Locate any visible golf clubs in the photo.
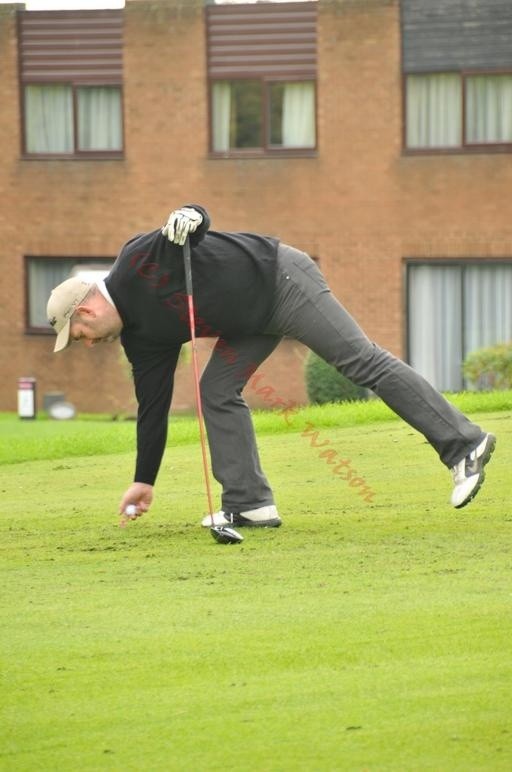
[182,233,242,543]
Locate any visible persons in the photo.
[47,203,497,532]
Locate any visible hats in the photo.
[47,277,95,353]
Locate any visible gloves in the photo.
[161,207,203,246]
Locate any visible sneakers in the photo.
[200,503,282,527]
[451,432,496,508]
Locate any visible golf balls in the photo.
[125,505,135,514]
